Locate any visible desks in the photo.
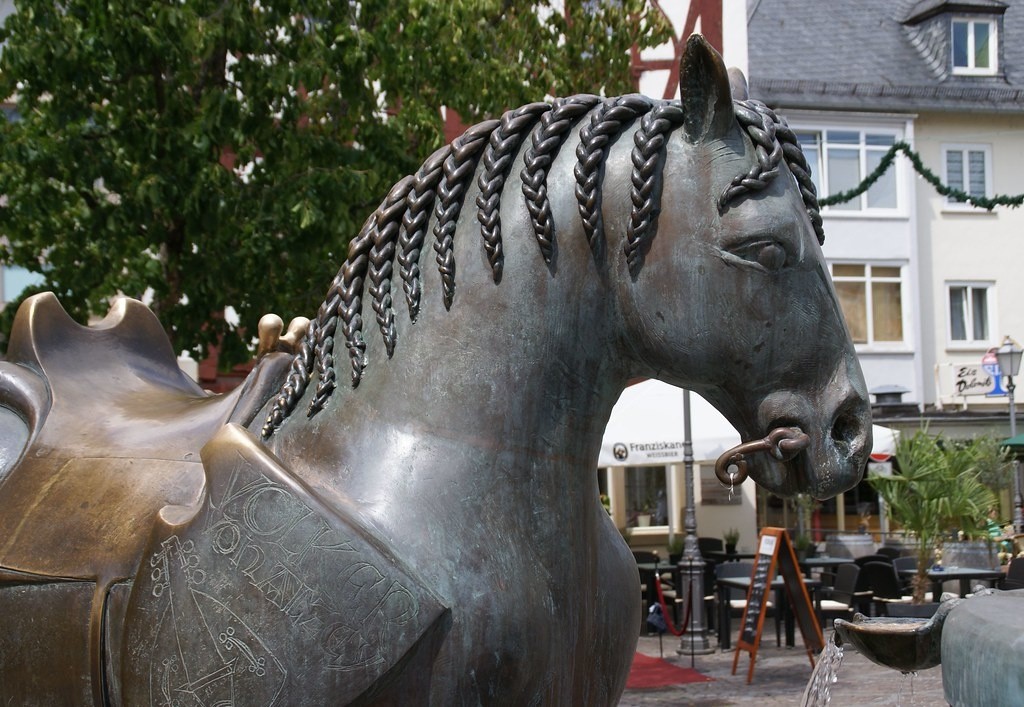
[898,565,1006,603]
[708,549,756,564]
[716,575,822,652]
[800,557,855,579]
[637,562,681,636]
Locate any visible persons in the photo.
[985,507,1015,554]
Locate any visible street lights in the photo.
[993,335,1024,540]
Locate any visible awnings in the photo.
[597,378,898,468]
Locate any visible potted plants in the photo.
[664,534,686,562]
[723,527,740,554]
[795,534,810,562]
[633,490,660,527]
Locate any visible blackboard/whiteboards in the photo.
[738,525,825,656]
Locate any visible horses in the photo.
[0,30,873,707]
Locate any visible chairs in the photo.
[631,537,1024,648]
